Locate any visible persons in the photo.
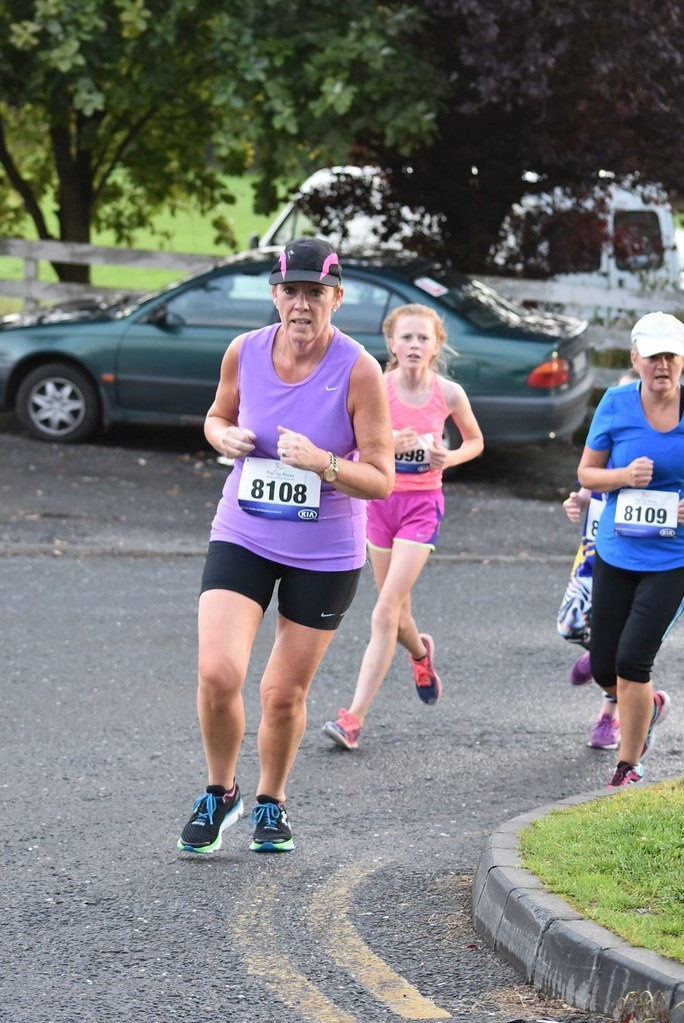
[575,312,684,788]
[176,236,397,855]
[320,303,485,750]
[555,368,643,751]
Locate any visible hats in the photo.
[269,237,342,287]
[631,310,684,358]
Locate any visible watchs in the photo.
[317,451,339,484]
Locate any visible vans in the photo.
[245,157,683,326]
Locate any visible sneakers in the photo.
[177,777,244,853]
[410,633,442,706]
[586,713,621,750]
[322,714,362,750]
[639,689,671,762]
[248,794,296,851]
[606,761,644,789]
[569,650,593,685]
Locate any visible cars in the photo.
[0,244,597,480]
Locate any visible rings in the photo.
[281,448,287,457]
